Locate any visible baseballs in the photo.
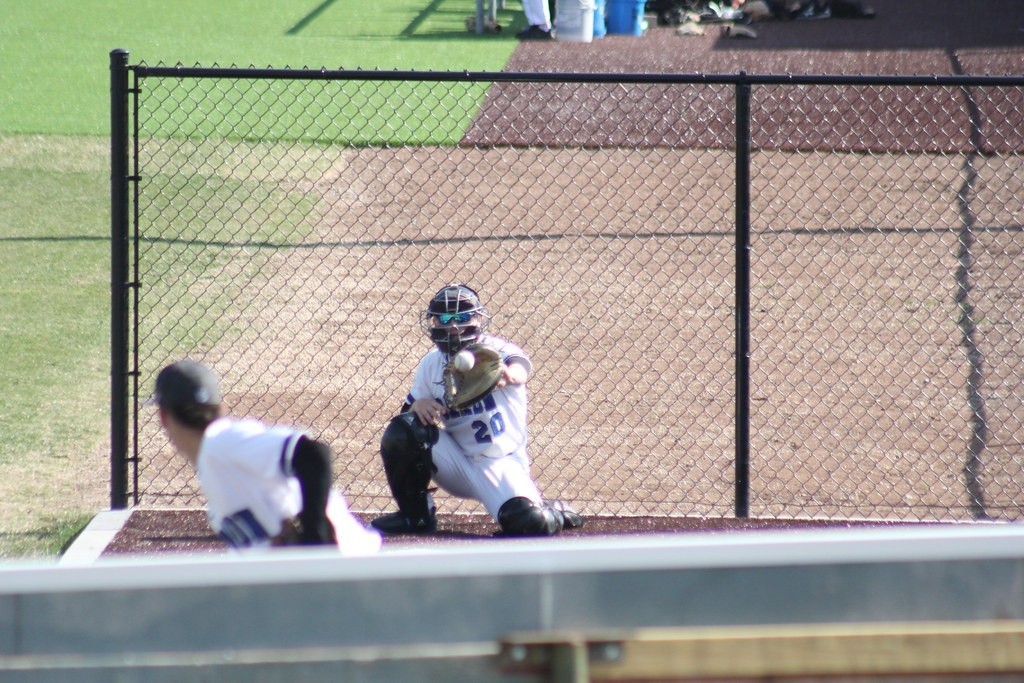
[455,349,475,372]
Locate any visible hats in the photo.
[156,361,220,410]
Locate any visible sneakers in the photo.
[550,499,583,527]
[370,511,437,533]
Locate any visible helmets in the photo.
[419,283,493,362]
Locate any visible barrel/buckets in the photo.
[552,0,648,42]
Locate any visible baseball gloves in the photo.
[441,347,503,410]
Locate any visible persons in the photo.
[513,0,554,39]
[155,360,383,551]
[369,282,584,538]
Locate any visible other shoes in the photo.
[515,25,551,38]
